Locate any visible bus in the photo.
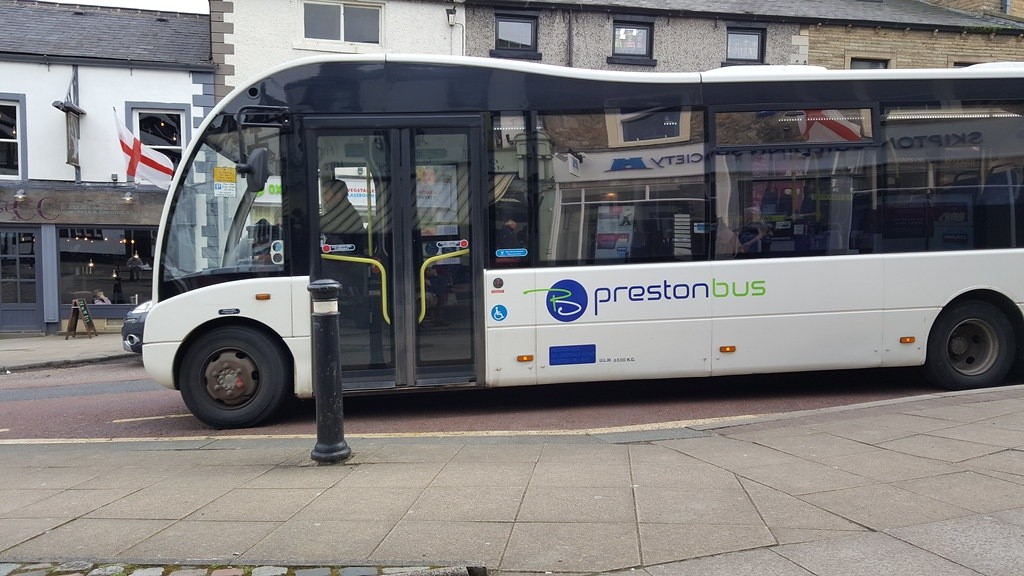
[142,54,1024,430]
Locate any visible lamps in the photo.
[122,192,134,204]
[13,189,26,203]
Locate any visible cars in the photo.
[121,300,152,354]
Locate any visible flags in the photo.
[798,110,861,143]
[114,114,174,190]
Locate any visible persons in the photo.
[318,179,382,332]
[618,208,632,227]
[663,114,674,137]
[498,219,524,258]
[728,204,772,258]
[93,289,111,305]
[426,161,474,325]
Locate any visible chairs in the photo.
[742,161,1024,259]
